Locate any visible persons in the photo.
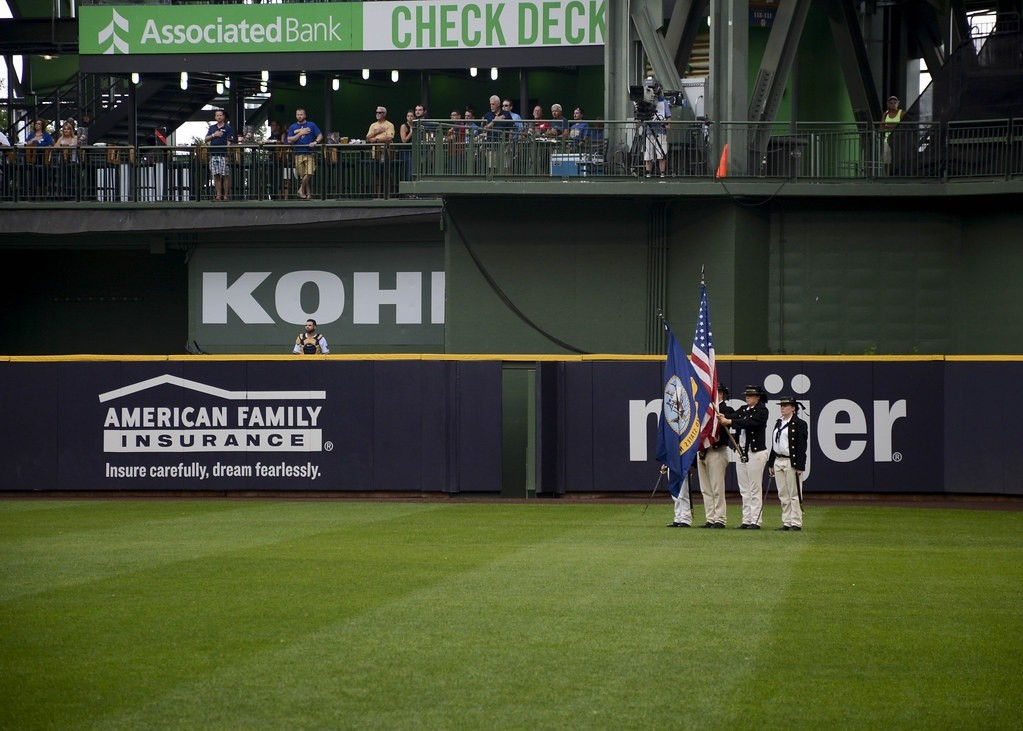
[447,95,591,178]
[292,319,330,354]
[715,385,769,529]
[643,92,672,177]
[366,106,396,199]
[205,111,236,202]
[0,118,81,200]
[288,108,323,198]
[879,95,907,176]
[696,383,736,529]
[399,104,436,197]
[767,396,808,531]
[658,463,693,528]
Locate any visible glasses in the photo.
[890,102,898,104]
[502,105,511,107]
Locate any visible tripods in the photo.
[627,117,673,175]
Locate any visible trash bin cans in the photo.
[767,137,808,177]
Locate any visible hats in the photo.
[888,96,899,102]
[741,384,762,395]
[777,396,795,405]
[717,383,726,390]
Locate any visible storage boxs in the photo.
[550,153,591,176]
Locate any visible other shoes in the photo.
[737,523,751,529]
[297,189,307,199]
[789,525,802,531]
[748,522,760,529]
[212,198,229,203]
[667,522,677,527]
[774,526,789,530]
[699,522,719,528]
[711,522,725,528]
[677,523,690,527]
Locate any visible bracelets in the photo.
[212,133,215,138]
[315,139,319,144]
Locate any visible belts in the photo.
[774,453,792,458]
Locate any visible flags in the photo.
[689,284,719,452]
[655,320,713,498]
